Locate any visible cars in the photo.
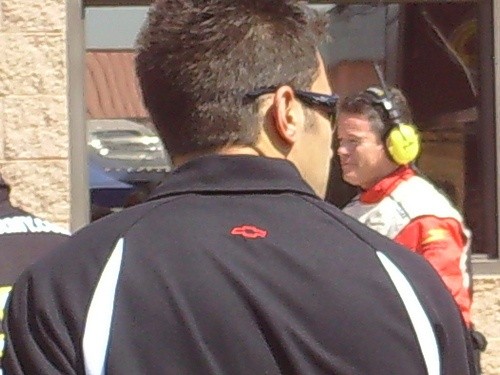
[86,117,165,171]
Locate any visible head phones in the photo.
[366,87,420,165]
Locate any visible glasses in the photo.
[245,86,338,131]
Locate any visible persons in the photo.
[1,0,481,375]
[333,79,475,331]
[0,170,72,360]
